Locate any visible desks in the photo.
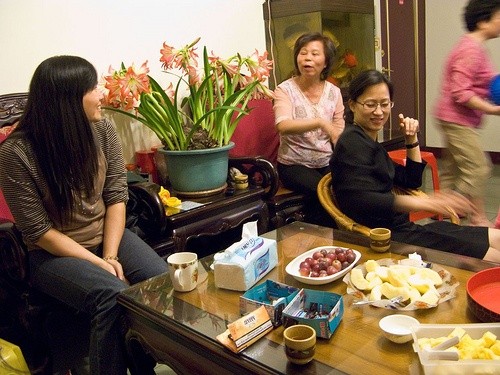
[117,222,500,375]
[164,183,270,253]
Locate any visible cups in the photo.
[166,252,198,292]
[234,175,248,189]
[369,228,391,253]
[283,325,317,365]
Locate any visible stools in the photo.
[388,149,444,222]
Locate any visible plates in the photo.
[285,245,361,284]
[342,257,460,311]
[466,267,500,323]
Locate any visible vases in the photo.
[157,142,234,197]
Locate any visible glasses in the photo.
[354,100,395,110]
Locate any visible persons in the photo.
[433,0,500,231]
[329,70,500,263]
[0,55,168,375]
[272,33,345,231]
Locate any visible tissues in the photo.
[209,220,279,291]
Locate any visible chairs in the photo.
[195,99,307,228]
[0,92,174,375]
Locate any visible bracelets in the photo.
[103,256,118,261]
[406,141,420,149]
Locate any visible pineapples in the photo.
[418,327,500,375]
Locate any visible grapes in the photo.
[299,248,356,277]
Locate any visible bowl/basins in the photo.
[412,322,500,375]
[379,313,420,344]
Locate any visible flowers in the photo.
[100,37,280,150]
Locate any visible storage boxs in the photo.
[262,0,376,110]
[240,279,344,340]
[411,322,500,375]
